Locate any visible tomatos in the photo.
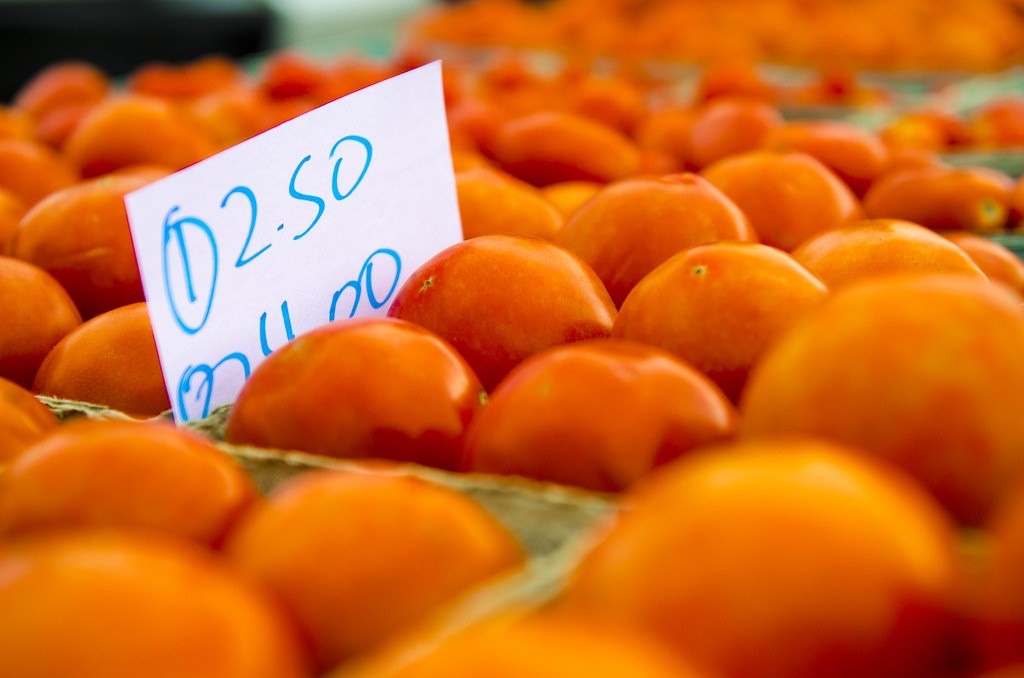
[0,0,1024,678]
